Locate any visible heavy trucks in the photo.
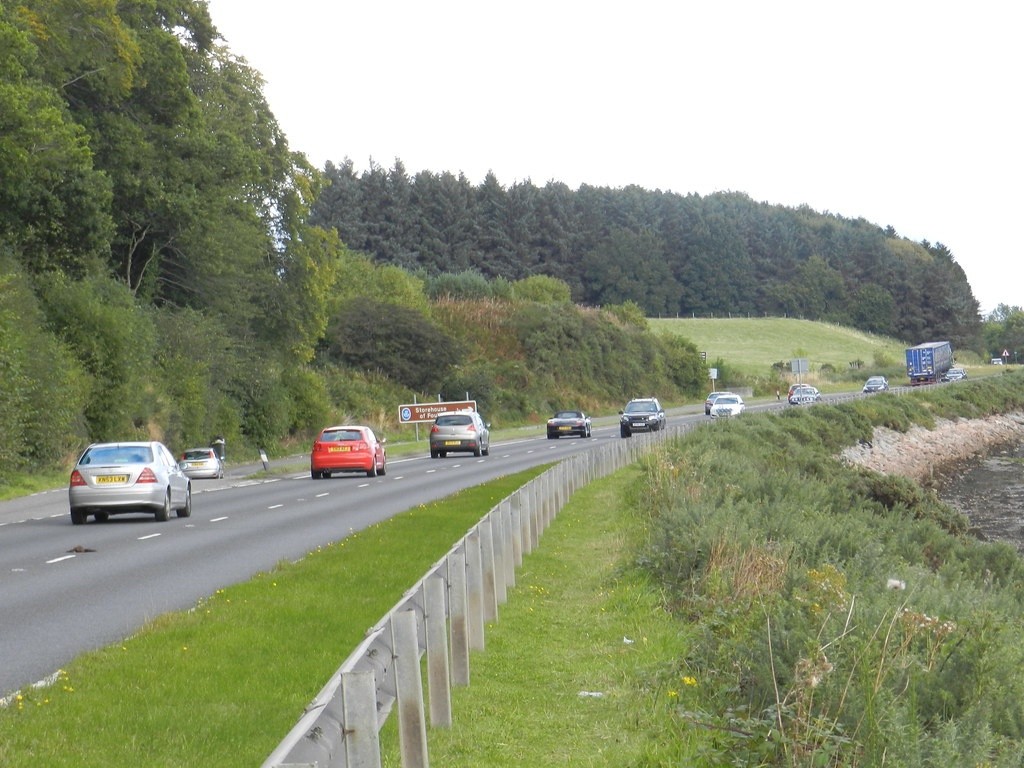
[906,340,955,387]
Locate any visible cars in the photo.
[944,368,968,382]
[709,395,746,419]
[704,391,736,415]
[788,383,824,405]
[429,411,492,459]
[179,446,224,480]
[67,440,191,525]
[546,410,593,439]
[309,424,387,479]
[862,375,889,393]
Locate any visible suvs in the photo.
[619,397,667,438]
